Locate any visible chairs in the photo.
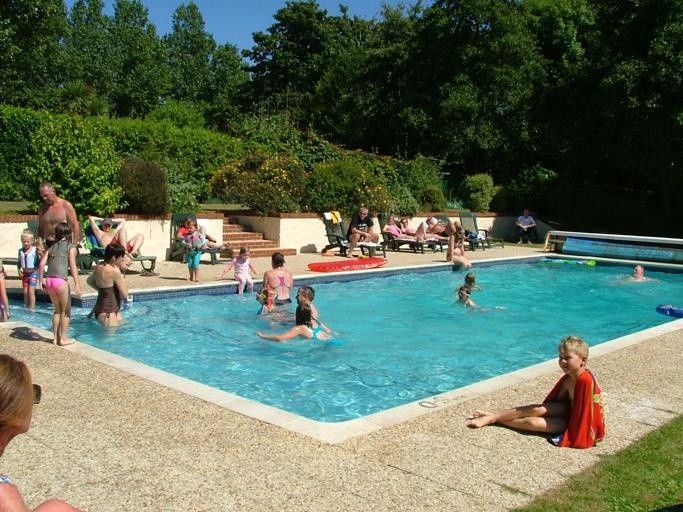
[168,212,234,265]
[21,219,100,278]
[314,206,539,259]
[77,215,157,280]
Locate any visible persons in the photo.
[456,287,473,306]
[461,273,481,291]
[447,228,471,273]
[0,261,10,324]
[628,264,646,279]
[36,182,79,258]
[0,354,82,512]
[346,206,485,257]
[467,335,605,448]
[515,210,537,244]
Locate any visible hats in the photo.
[102,218,113,225]
